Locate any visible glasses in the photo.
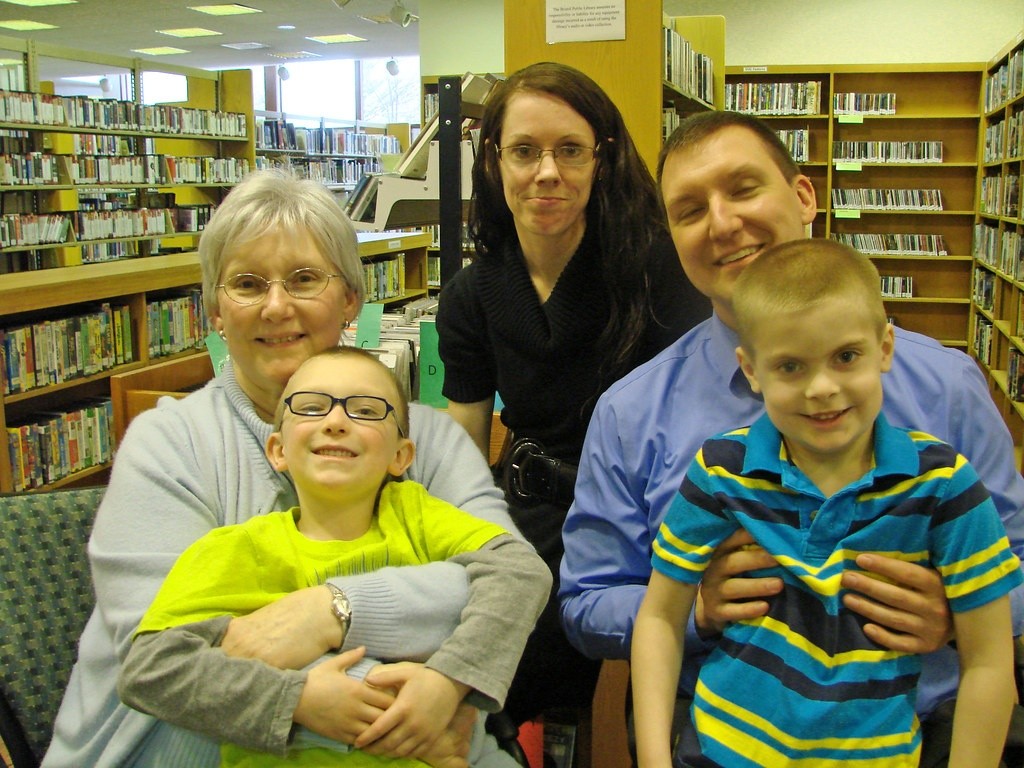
[275,392,406,437]
[493,142,600,166]
[215,267,344,304]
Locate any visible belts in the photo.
[493,429,575,506]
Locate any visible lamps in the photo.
[332,0,351,9]
[98,76,114,92]
[277,63,289,80]
[389,2,415,28]
[385,55,402,77]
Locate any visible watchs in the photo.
[325,583,352,646]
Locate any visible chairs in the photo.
[0,472,529,768]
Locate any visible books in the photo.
[662,29,1024,402]
[0,71,505,497]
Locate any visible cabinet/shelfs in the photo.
[0,0,1024,495]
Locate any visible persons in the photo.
[436,61,704,768]
[39,166,553,768]
[557,109,1024,768]
